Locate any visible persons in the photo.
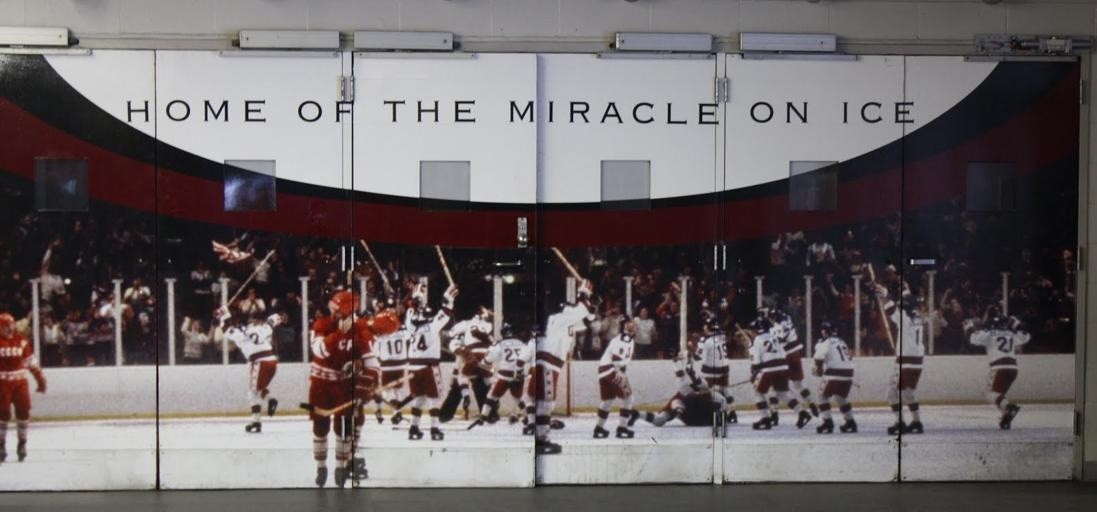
[4,206,1077,492]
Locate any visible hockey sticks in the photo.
[851,263,895,351]
[300,374,416,417]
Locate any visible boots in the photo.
[18,443,26,456]
[432,427,444,436]
[410,425,423,435]
[375,410,402,422]
[0,445,7,457]
[247,422,261,431]
[268,399,277,415]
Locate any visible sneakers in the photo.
[479,415,565,432]
[315,459,365,486]
[593,408,641,438]
[721,405,1016,434]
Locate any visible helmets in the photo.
[898,293,916,314]
[819,321,833,335]
[411,304,542,338]
[704,317,720,332]
[991,315,1007,329]
[374,311,399,337]
[0,313,15,339]
[768,306,783,322]
[618,314,636,338]
[328,290,359,319]
[751,317,772,333]
